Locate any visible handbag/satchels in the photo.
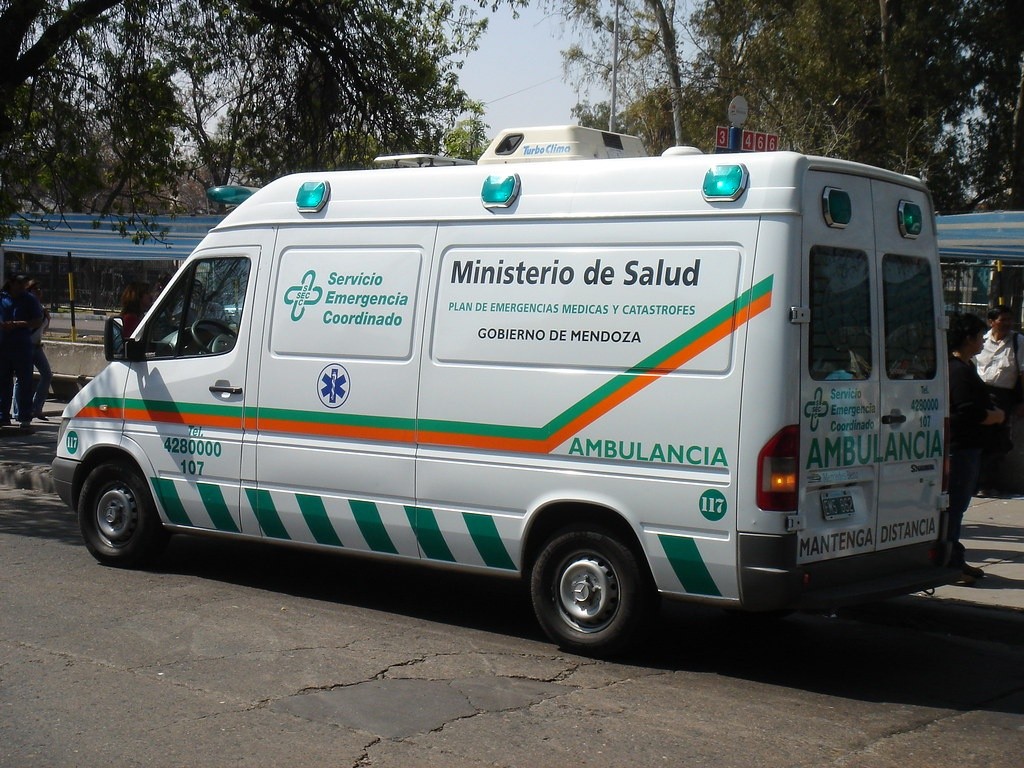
[1010,375,1024,404]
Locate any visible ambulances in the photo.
[52,128,953,661]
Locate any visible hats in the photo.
[25,280,40,289]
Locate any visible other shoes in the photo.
[0,420,11,425]
[20,422,35,434]
[32,409,49,421]
[990,489,1000,497]
[977,489,986,497]
[955,562,984,581]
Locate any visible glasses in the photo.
[35,287,41,290]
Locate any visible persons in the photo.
[120,271,195,343]
[13,277,52,421]
[970,304,1024,499]
[945,312,1006,585]
[0,272,44,434]
[818,349,870,537]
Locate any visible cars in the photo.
[220,292,246,319]
